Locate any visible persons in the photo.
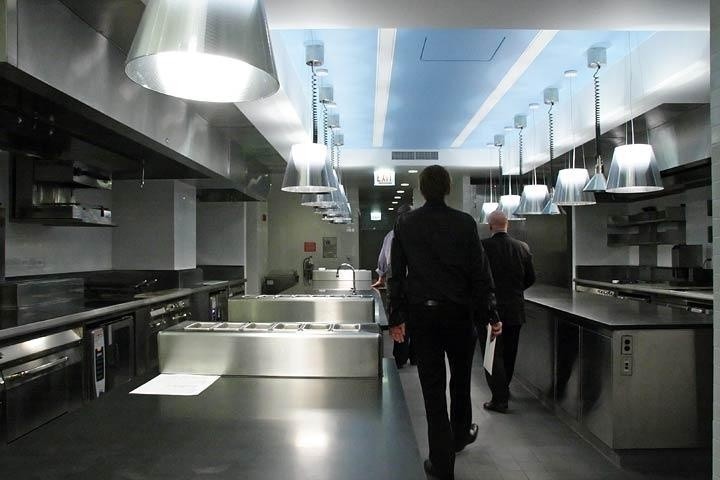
[475,207,534,416]
[384,161,504,480]
[371,204,419,369]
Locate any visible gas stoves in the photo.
[610,274,674,288]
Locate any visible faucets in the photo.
[336,262,356,292]
[136,279,158,293]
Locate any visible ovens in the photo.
[0,323,87,446]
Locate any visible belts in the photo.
[426,299,445,307]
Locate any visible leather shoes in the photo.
[454,423,479,452]
[424,458,433,476]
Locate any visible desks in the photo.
[512,282,713,474]
[0,356,431,479]
[277,281,391,356]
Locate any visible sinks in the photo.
[134,286,190,299]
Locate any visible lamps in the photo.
[122,0,282,104]
[477,46,665,225]
[279,37,338,195]
[297,83,352,227]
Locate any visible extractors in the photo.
[10,159,115,228]
[605,207,687,246]
